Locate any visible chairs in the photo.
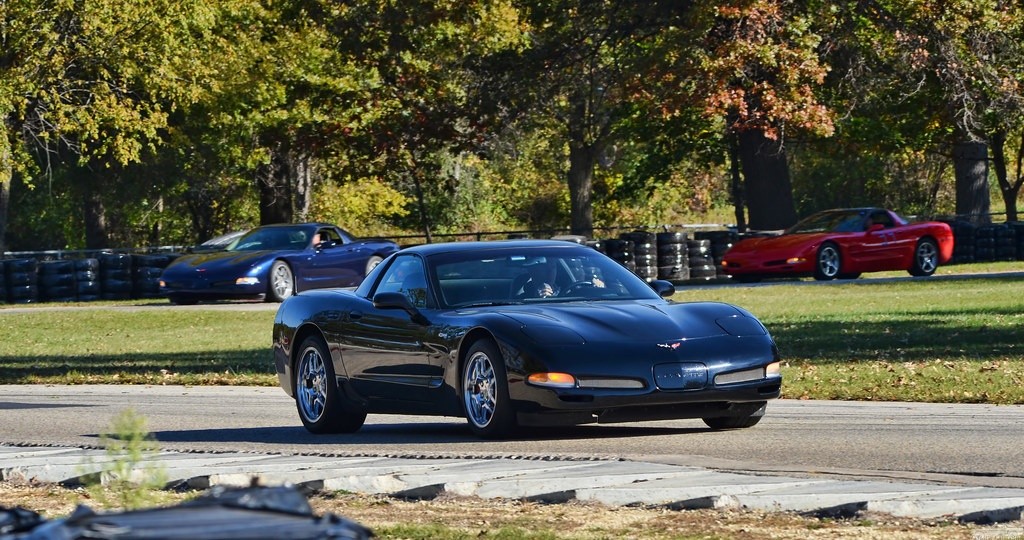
[320,231,332,242]
[400,272,428,308]
[509,273,534,299]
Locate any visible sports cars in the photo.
[158,222,401,306]
[721,207,954,281]
[272,239,783,440]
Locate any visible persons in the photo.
[517,260,605,298]
[311,233,327,249]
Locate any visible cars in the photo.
[163,231,248,261]
